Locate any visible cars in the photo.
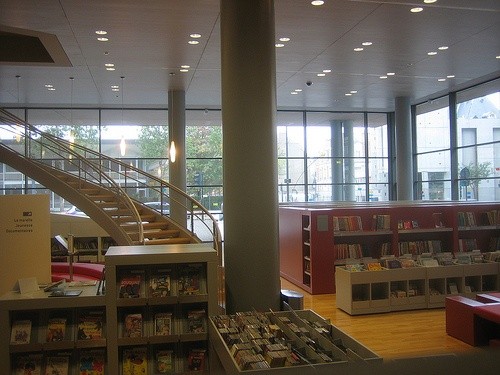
[67,205,88,216]
[144,202,170,216]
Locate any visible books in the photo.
[459,237,500,252]
[398,240,442,256]
[212,311,347,370]
[303,215,310,287]
[345,250,500,271]
[458,210,497,227]
[334,243,391,259]
[391,282,471,298]
[333,215,390,230]
[119,266,208,375]
[10,313,106,375]
[398,220,446,229]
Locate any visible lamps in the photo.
[169,73,176,163]
[69,77,75,151]
[120,76,126,155]
[16,76,21,142]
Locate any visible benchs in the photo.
[51,259,105,281]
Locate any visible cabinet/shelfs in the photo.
[0,247,217,375]
[279,204,500,295]
[208,310,383,375]
[335,258,500,316]
